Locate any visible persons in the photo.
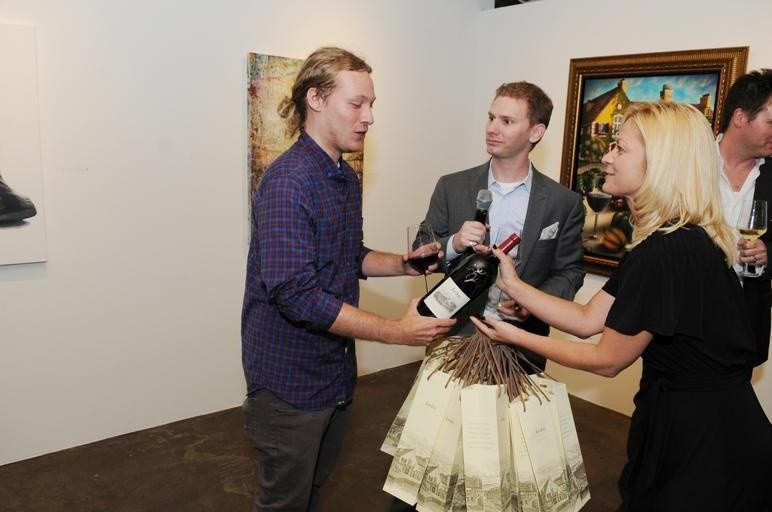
[389,80,586,510]
[469,101,771,510]
[699,67,771,382]
[242,46,458,512]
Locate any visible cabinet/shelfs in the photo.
[417,232,521,321]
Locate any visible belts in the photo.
[584,174,612,243]
[733,201,767,279]
[408,225,438,295]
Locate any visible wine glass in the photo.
[752,256,757,264]
[470,241,473,247]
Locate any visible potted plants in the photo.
[559,45,749,278]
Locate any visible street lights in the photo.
[378,335,593,512]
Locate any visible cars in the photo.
[483,257,522,319]
